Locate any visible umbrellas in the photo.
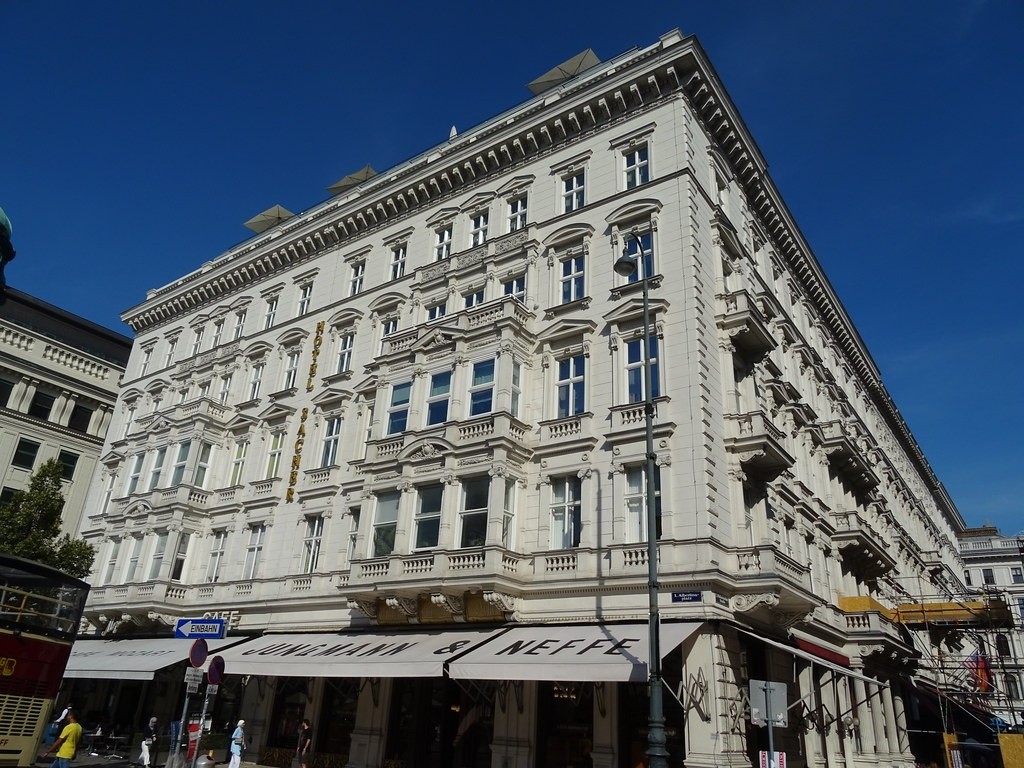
[327,164,377,194]
[524,48,600,95]
[243,204,295,233]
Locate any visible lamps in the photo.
[805,712,818,735]
[825,713,835,737]
[841,716,853,739]
[848,718,860,739]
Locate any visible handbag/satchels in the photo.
[145,738,153,745]
[235,738,242,743]
[292,752,299,768]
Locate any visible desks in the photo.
[104,736,129,760]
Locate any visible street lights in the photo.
[614,232,670,768]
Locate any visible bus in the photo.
[0,553,91,768]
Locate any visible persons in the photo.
[42,710,82,768]
[138,717,158,768]
[54,704,74,752]
[298,719,312,768]
[228,720,246,768]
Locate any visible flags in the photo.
[962,647,995,700]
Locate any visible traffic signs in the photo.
[175,619,224,639]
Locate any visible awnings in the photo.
[917,679,1013,729]
[62,620,706,711]
